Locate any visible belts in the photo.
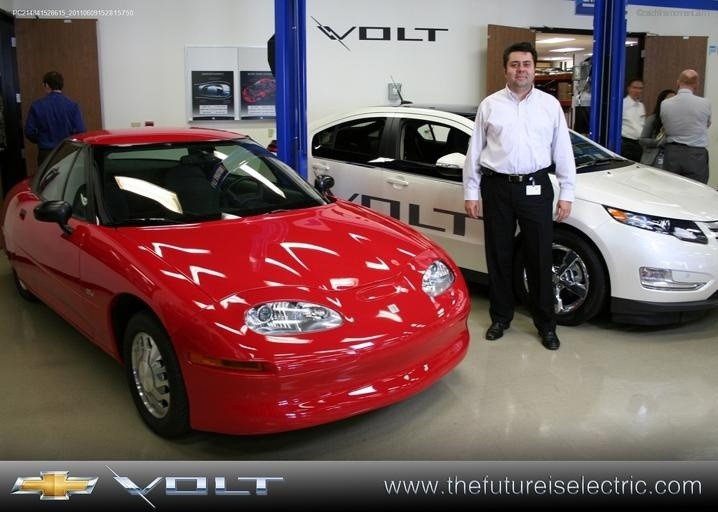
[665,142,688,147]
[480,165,548,183]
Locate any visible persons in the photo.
[24,71,84,167]
[639,70,711,185]
[463,42,576,350]
[622,78,646,162]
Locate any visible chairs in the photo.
[75,165,222,218]
[334,126,468,157]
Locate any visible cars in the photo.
[241,80,275,104]
[193,80,232,102]
[255,73,718,328]
[0,125,474,440]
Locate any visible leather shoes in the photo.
[538,328,560,350]
[486,323,510,341]
